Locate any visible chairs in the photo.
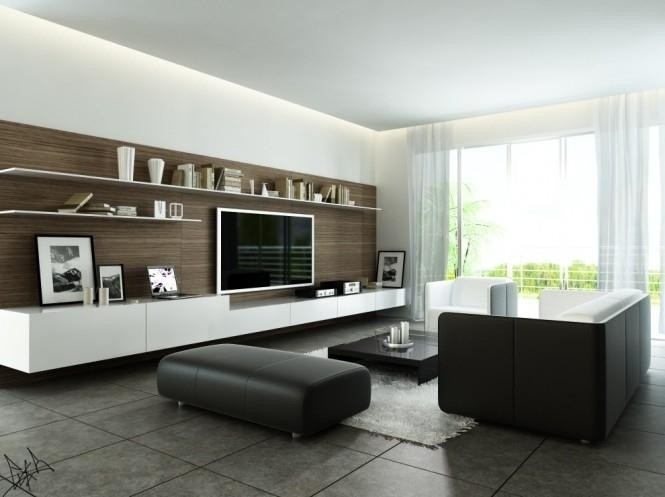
[423,277,519,332]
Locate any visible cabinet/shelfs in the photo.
[292,289,406,327]
[2,298,147,374]
[143,292,291,354]
[0,168,384,222]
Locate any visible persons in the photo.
[52,268,81,290]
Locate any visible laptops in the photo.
[146,265,202,300]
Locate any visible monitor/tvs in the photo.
[216,207,315,294]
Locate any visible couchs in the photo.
[437,290,652,444]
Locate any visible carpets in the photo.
[305,347,480,448]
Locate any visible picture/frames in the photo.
[376,250,405,288]
[98,264,127,303]
[36,234,99,307]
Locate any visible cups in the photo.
[83,286,93,306]
[98,288,110,306]
[388,320,410,343]
[147,158,165,184]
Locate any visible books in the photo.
[172,162,244,193]
[57,192,137,216]
[276,175,350,204]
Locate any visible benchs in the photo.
[158,343,372,439]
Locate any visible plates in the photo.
[384,341,415,348]
